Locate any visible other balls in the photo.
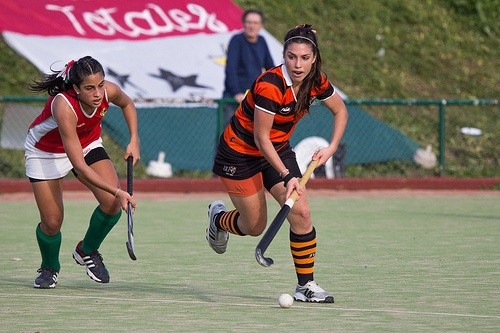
[278,294,294,308]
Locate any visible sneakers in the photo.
[72,240,110,283]
[205,200,229,254]
[293,280,334,303]
[33,267,60,289]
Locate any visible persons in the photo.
[205,24,349,303]
[221,9,276,125]
[24,55,141,289]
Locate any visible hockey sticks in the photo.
[126,155,137,261]
[255,156,320,267]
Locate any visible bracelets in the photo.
[279,169,295,187]
[114,187,121,198]
